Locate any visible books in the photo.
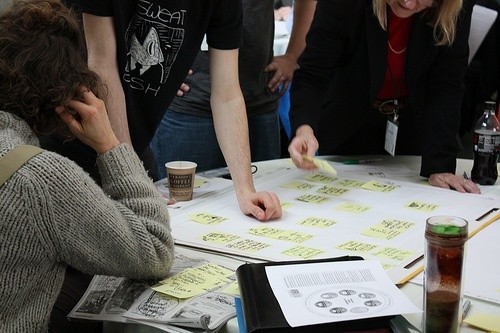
[233,255,409,333]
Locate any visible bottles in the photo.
[423,215,468,333]
[471,101,500,184]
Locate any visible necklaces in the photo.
[388,41,407,54]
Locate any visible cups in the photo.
[165,160,198,201]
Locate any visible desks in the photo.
[102,155,500,333]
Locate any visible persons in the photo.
[75,0,281,221]
[151,0,316,184]
[288,0,481,194]
[0,0,174,333]
